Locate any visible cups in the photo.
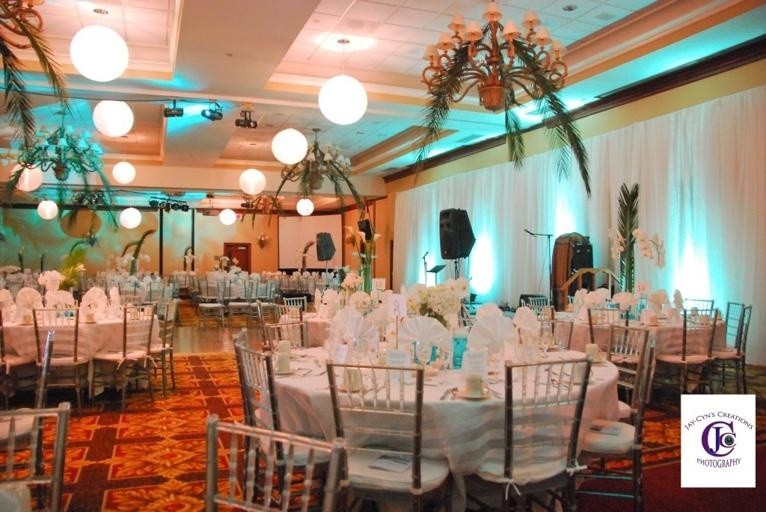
[276,320,602,400]
[21,303,154,325]
[592,307,724,326]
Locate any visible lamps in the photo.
[164,99,183,117]
[148,194,189,211]
[235,111,257,128]
[281,126,353,191]
[201,102,223,120]
[13,108,103,181]
[422,1,568,113]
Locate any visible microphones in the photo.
[524,228,535,236]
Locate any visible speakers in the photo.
[358,219,372,241]
[439,208,476,259]
[571,245,594,287]
[316,233,336,261]
